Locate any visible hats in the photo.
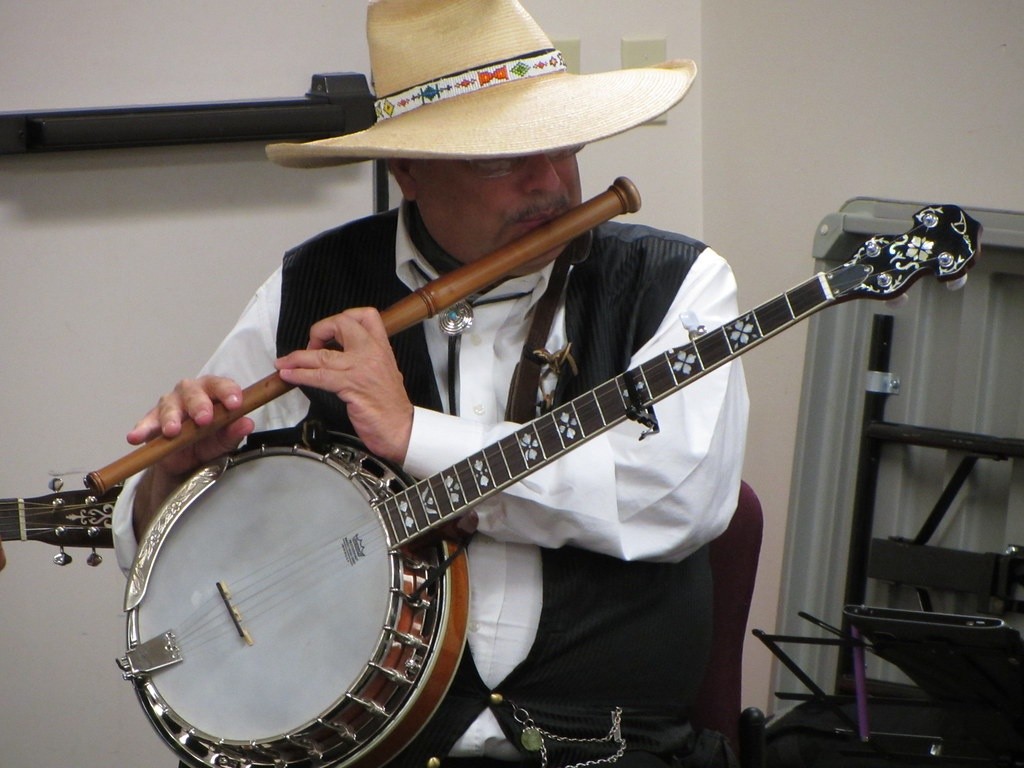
[264,0,698,169]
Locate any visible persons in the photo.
[112,0,750,768]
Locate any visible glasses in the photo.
[466,145,583,179]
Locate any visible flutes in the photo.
[79,177,644,496]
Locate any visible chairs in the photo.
[688,476,765,768]
[762,314,1023,768]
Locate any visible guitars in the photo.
[117,202,984,768]
[1,471,123,567]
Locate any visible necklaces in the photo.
[409,259,534,335]
